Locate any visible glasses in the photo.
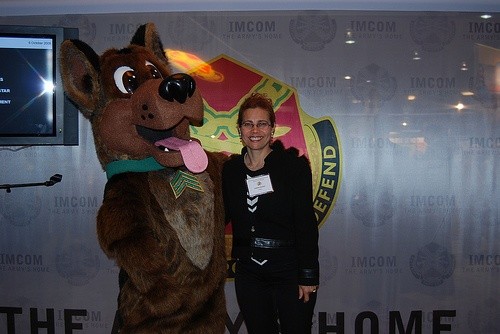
[240,120,272,130]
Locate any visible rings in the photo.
[312,289,316,292]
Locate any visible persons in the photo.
[220,92,320,334]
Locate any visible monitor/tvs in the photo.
[0,25,79,146]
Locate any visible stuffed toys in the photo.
[58,21,311,334]
